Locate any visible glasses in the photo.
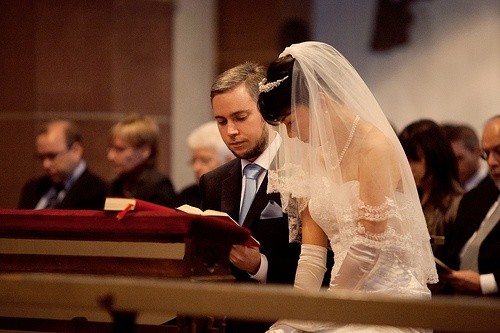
[38,148,71,160]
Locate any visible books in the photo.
[176,204,261,248]
[103,196,169,211]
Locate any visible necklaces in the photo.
[328,114,361,169]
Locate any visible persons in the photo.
[107,112,176,209]
[433,115,500,298]
[445,124,490,193]
[199,62,334,333]
[176,120,236,208]
[397,119,468,254]
[16,118,110,211]
[257,41,439,333]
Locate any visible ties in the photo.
[239,163,265,226]
[457,201,500,270]
